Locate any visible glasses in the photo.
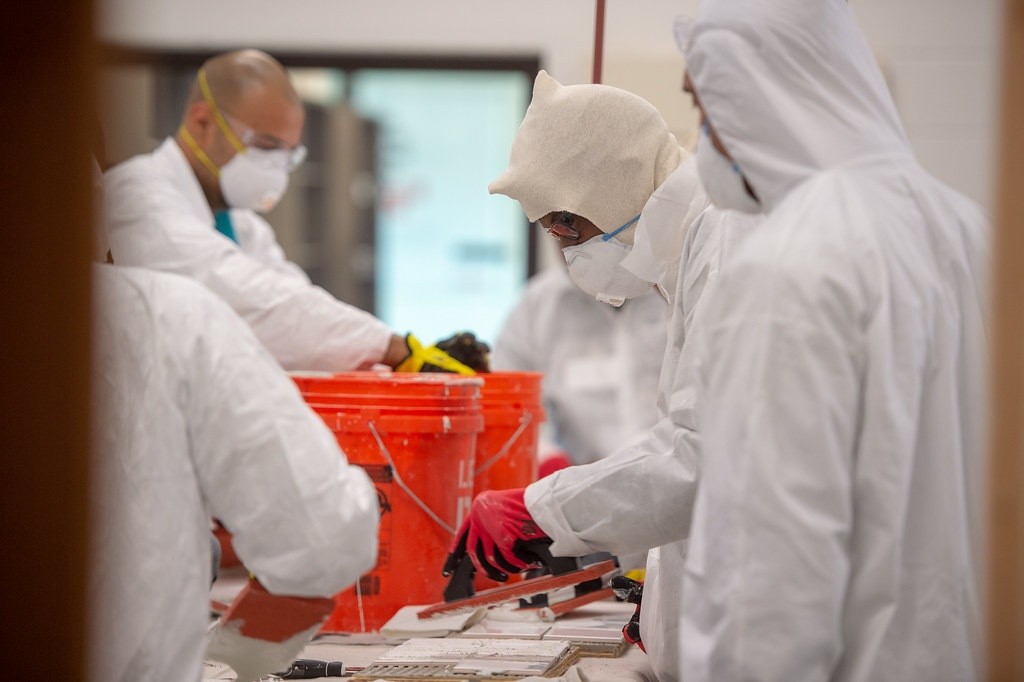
[543,211,582,240]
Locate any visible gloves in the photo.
[448,487,549,583]
[391,331,491,377]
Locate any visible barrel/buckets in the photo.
[465,372,547,592]
[288,371,485,636]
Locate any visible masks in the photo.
[693,118,760,215]
[560,213,654,301]
[214,145,292,215]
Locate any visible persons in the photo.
[108,49,492,375]
[486,268,668,482]
[679,1,991,682]
[85,265,378,682]
[439,73,765,606]
